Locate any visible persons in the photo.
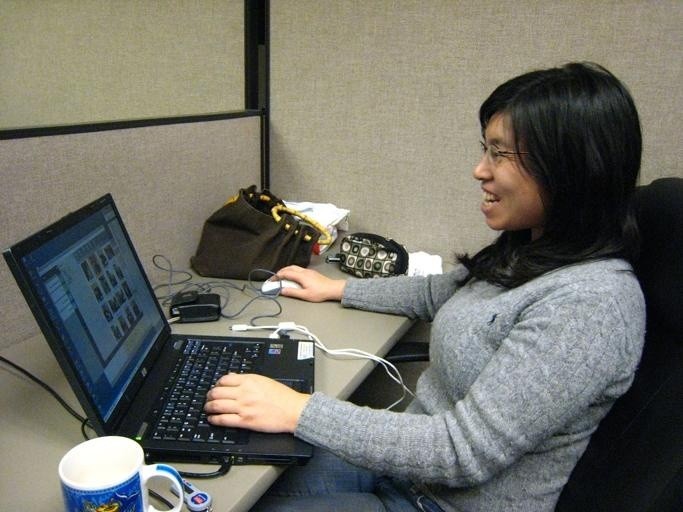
[201,61,649,511]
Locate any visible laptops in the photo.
[2,191,315,467]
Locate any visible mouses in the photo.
[260,277,301,297]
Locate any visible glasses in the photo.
[478,139,523,166]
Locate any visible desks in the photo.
[0,244,420,512]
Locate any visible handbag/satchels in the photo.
[189,184,332,282]
[339,233,410,280]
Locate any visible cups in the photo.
[57,434,186,512]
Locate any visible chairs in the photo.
[554,177,682,512]
